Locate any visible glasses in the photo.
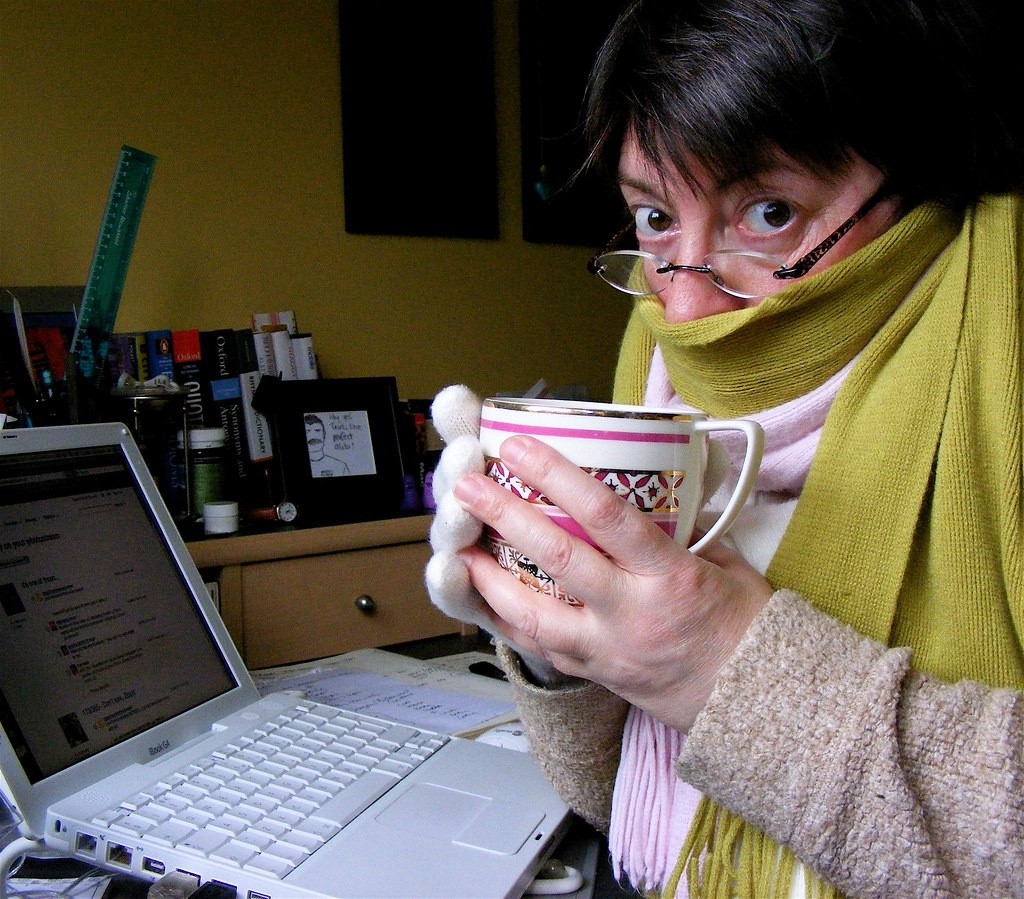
[586,181,887,298]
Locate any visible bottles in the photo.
[178,430,228,513]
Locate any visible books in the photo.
[113,308,314,525]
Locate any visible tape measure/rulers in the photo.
[64,145,159,393]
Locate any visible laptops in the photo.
[0,421,578,899]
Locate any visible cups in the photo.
[479,396,765,608]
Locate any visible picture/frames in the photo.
[0,286,86,415]
[251,374,408,524]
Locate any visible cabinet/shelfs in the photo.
[182,510,478,666]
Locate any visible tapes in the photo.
[203,501,239,518]
[204,516,238,533]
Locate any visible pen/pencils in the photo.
[43,371,55,410]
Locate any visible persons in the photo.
[425,0,1024,898]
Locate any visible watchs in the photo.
[252,501,297,522]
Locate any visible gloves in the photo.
[425,385,731,686]
